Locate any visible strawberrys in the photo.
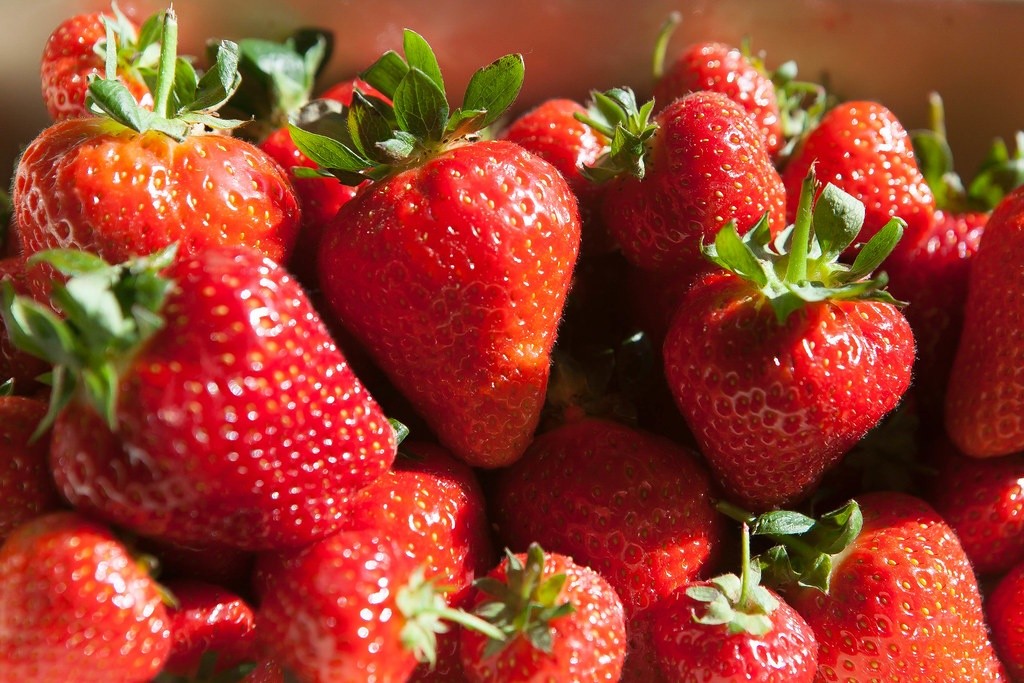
[0,0,1024,683]
